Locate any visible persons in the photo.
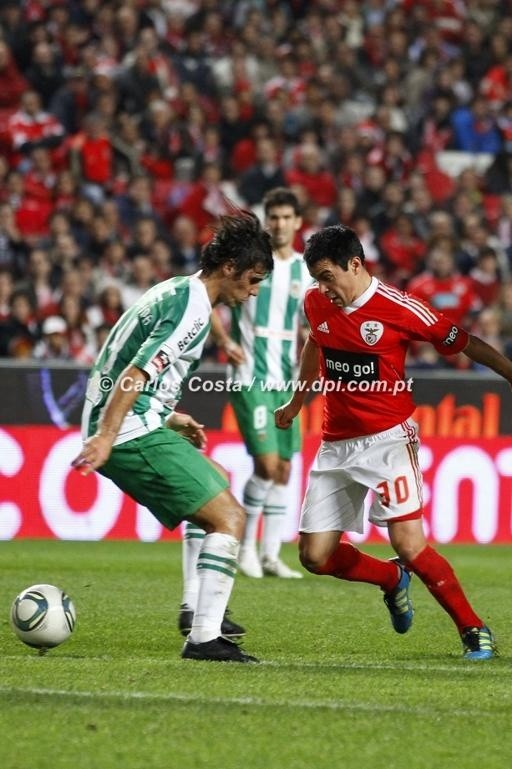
[211,186,323,582]
[63,209,275,665]
[271,225,510,664]
[1,0,511,372]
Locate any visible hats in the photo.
[42,315,69,335]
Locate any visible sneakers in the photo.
[461,622,495,661]
[239,544,264,580]
[383,556,413,634]
[261,555,303,579]
[182,633,258,666]
[177,603,247,636]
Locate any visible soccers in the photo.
[10,583,77,649]
